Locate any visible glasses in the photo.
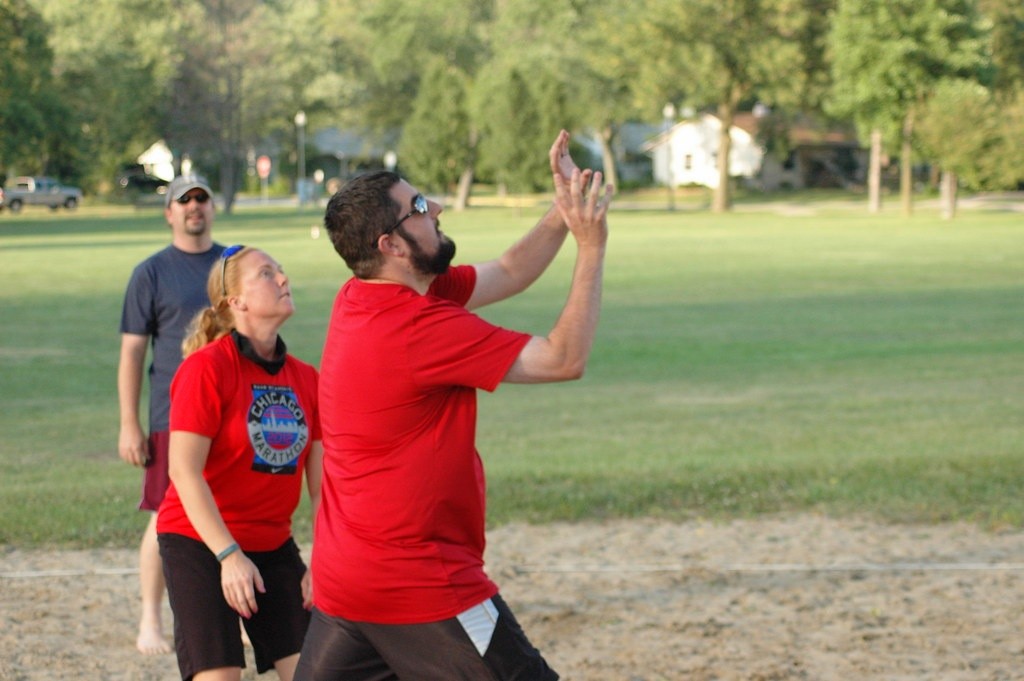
[371,193,429,249]
[176,194,209,203]
[220,245,244,297]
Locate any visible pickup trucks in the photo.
[0,175,83,211]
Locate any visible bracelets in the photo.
[216,543,239,562]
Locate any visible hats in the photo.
[165,175,213,208]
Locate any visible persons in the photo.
[117,176,228,655]
[156,246,323,681]
[291,129,614,681]
